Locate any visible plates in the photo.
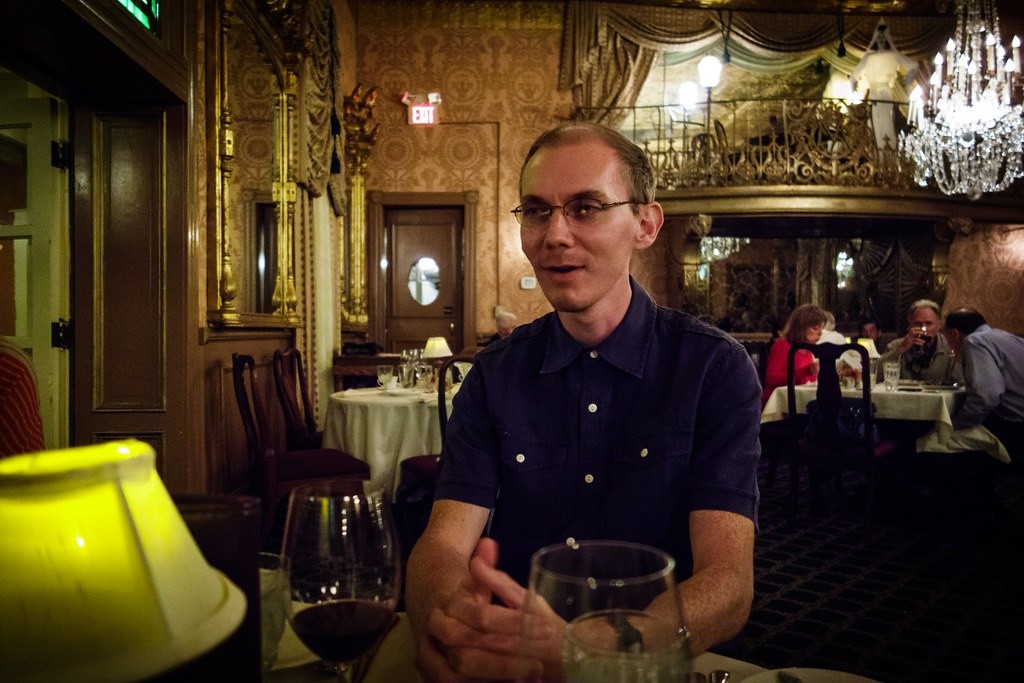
[740,667,877,683]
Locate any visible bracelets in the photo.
[608,614,643,655]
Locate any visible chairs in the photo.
[230,345,479,594]
[743,337,1024,561]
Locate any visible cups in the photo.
[259,477,400,683]
[377,349,452,394]
[518,539,691,683]
[857,359,901,392]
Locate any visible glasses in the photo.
[509,196,647,228]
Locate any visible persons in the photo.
[762,300,962,496]
[940,310,1024,541]
[482,313,515,347]
[406,121,760,683]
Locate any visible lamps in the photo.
[0,437,247,683]
[895,0,1024,196]
[717,9,731,63]
[837,12,847,58]
[422,336,453,380]
[401,93,443,129]
[697,54,723,185]
[853,338,880,377]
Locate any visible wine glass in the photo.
[914,326,932,359]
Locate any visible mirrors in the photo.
[205,1,302,329]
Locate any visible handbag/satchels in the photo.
[804,396,881,448]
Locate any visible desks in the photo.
[760,379,964,447]
[323,386,460,504]
